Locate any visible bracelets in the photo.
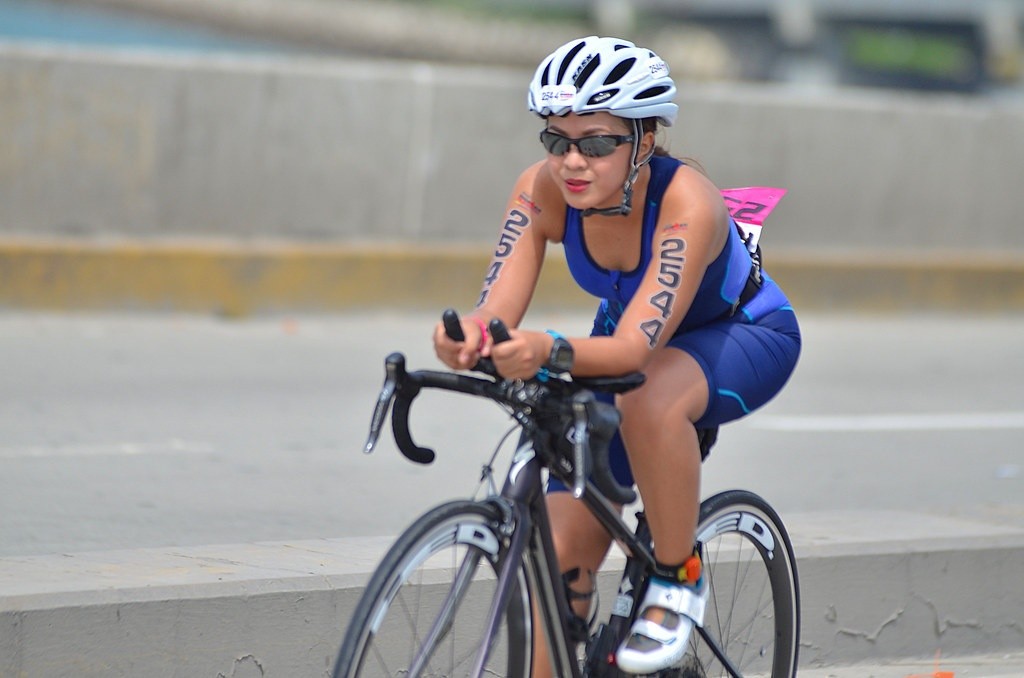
[464,316,488,351]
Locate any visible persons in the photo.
[433,35,801,678]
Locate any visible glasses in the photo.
[538,127,635,158]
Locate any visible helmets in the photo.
[527,36,678,127]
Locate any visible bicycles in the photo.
[331,308,803,678]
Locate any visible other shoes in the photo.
[616,568,708,673]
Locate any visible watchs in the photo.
[537,330,574,382]
